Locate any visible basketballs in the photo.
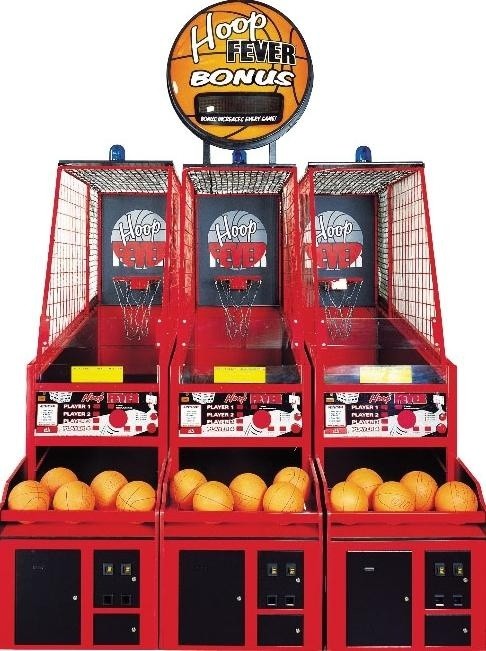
[170,467,312,526]
[7,467,156,525]
[332,467,478,527]
